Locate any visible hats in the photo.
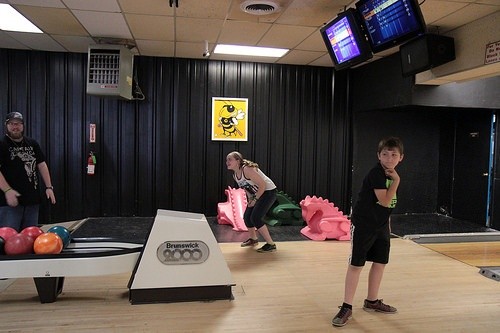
[4,112,23,125]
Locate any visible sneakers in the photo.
[241,238,258,247]
[332,306,352,326]
[363,299,398,314]
[256,244,277,252]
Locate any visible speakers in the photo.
[398,33,456,78]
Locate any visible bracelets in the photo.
[45,186,54,189]
[4,188,12,194]
[254,198,258,202]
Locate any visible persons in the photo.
[226,151,277,253]
[0,112,55,280]
[331,137,404,326]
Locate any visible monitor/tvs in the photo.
[319,0,426,71]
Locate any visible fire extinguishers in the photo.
[86,149,98,176]
